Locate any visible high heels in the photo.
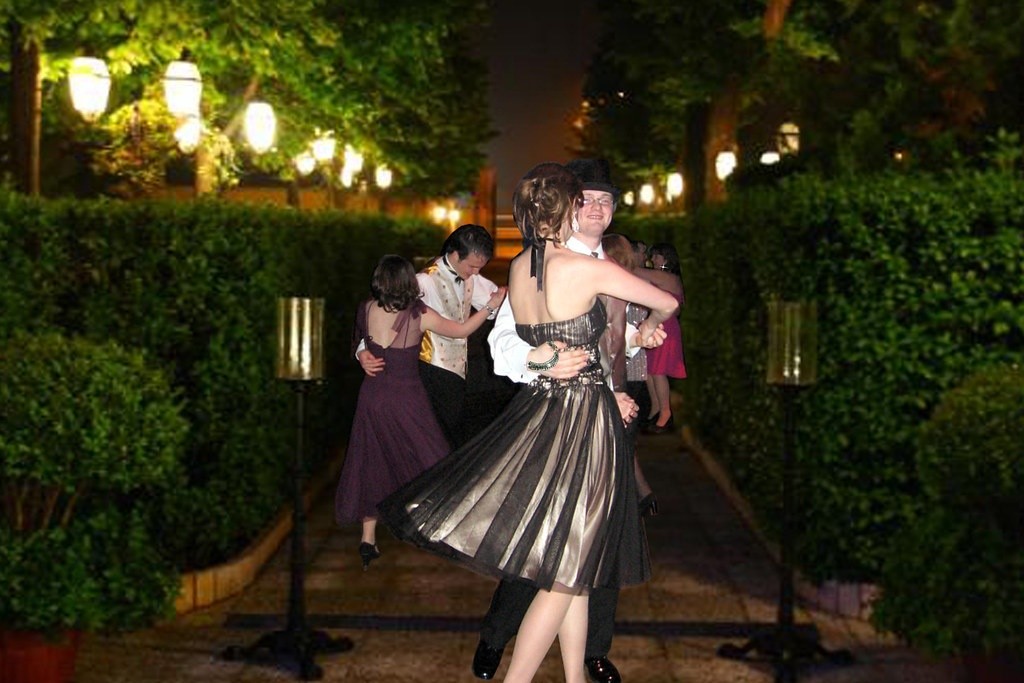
[636,410,673,436]
[358,542,380,571]
[638,493,658,517]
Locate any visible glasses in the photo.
[578,192,614,206]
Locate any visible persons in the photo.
[339,254,511,572]
[354,224,507,449]
[375,158,686,683]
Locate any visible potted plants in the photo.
[869,366,1024,683]
[0,325,195,683]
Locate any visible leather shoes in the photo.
[472,639,504,679]
[584,655,621,683]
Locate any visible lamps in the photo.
[714,146,738,182]
[68,43,113,113]
[272,293,327,395]
[245,94,278,156]
[761,297,822,394]
[159,46,202,116]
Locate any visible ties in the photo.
[591,252,598,259]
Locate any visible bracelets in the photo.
[484,304,495,315]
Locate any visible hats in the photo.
[563,158,622,196]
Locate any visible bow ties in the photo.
[443,255,465,286]
